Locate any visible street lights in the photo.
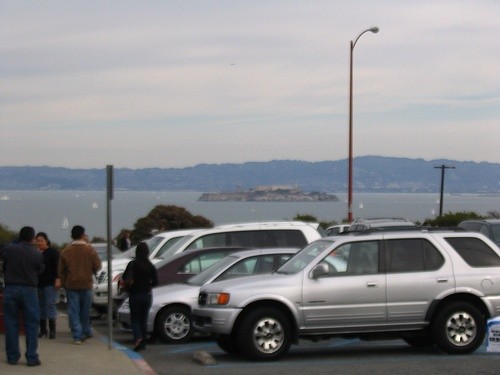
[348,27,380,224]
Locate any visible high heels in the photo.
[38,329,48,339]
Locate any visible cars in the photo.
[116,247,302,346]
[119,247,254,331]
[324,217,464,235]
[457,219,500,249]
[88,242,122,262]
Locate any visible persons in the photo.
[121,242,159,351]
[55,225,102,344]
[35,232,59,339]
[0,226,45,367]
[120,231,132,250]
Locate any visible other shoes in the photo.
[74,339,82,345]
[27,358,41,367]
[86,328,94,336]
[133,336,144,351]
[7,352,22,364]
[49,329,56,339]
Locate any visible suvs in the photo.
[189,231,500,361]
[147,222,323,264]
[93,228,209,314]
